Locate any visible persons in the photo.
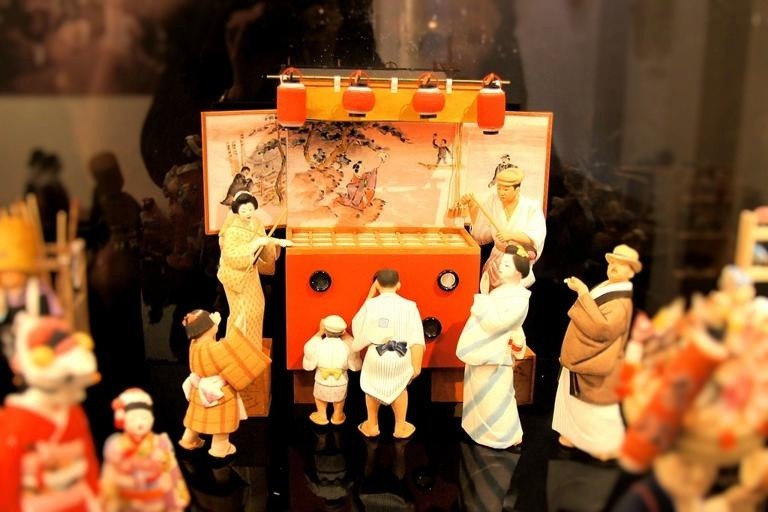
[139,0,390,189]
[97,385,192,510]
[0,309,102,511]
[552,243,644,461]
[351,268,427,440]
[455,243,542,451]
[216,189,282,352]
[459,166,549,360]
[301,313,364,426]
[87,147,144,359]
[177,307,275,460]
